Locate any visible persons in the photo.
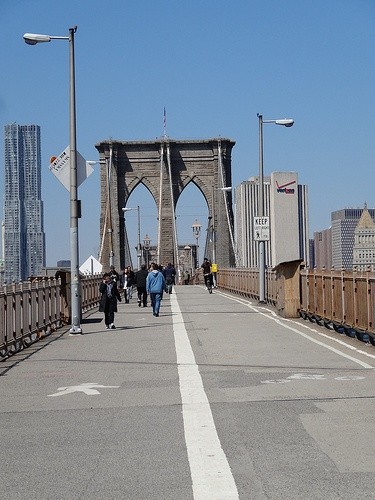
[146,263,165,317]
[99,265,122,329]
[201,258,212,290]
[119,266,135,304]
[136,264,149,307]
[162,263,176,294]
[184,271,190,285]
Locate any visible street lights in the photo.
[257,118,295,302]
[210,184,233,290]
[22,31,83,336]
[86,159,115,271]
[121,206,142,272]
[192,219,203,272]
[141,233,152,271]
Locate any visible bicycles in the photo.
[199,272,214,295]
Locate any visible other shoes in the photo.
[153,313,155,316]
[130,296,132,298]
[138,304,141,306]
[125,300,129,304]
[156,313,159,316]
[208,287,211,290]
[209,290,212,294]
[143,304,147,307]
[166,291,168,293]
[169,292,172,294]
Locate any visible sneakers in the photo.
[109,323,115,328]
[105,325,109,328]
